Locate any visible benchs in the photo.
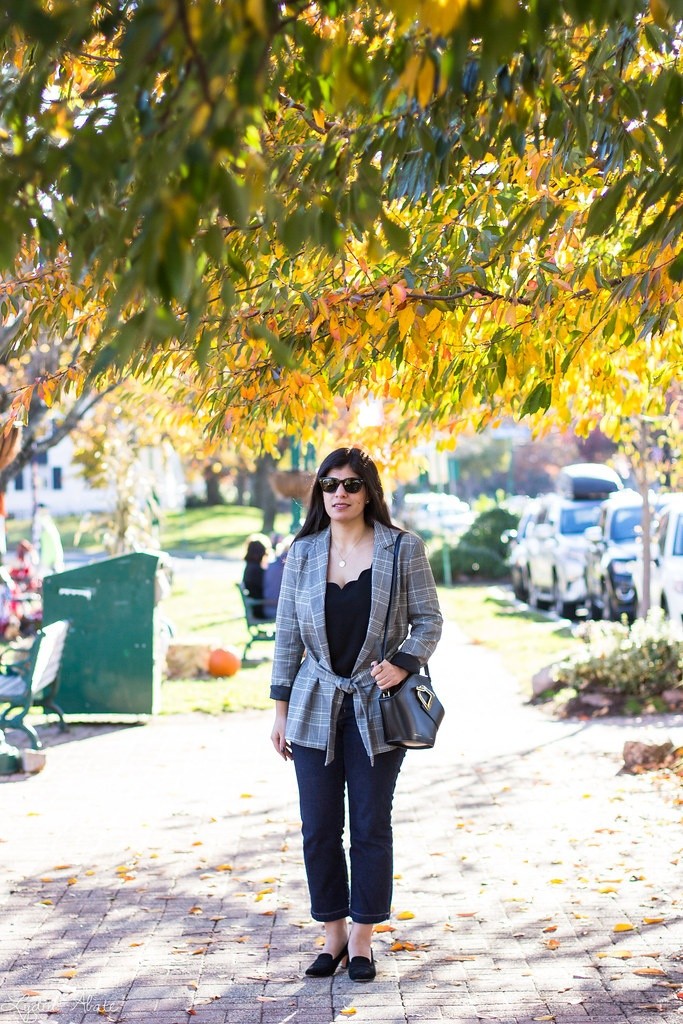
[0,620,72,749]
[235,582,276,663]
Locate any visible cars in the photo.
[404,493,537,534]
[635,494,683,626]
[503,501,535,599]
[584,503,652,615]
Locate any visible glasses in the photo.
[319,478,366,493]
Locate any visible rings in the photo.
[379,664,383,671]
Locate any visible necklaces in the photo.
[333,534,362,567]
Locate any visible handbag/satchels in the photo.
[378,673,445,750]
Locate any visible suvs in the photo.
[524,501,606,617]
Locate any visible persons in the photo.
[36,504,64,574]
[263,534,296,618]
[243,534,272,618]
[269,447,443,982]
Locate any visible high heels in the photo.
[349,947,376,981]
[305,941,352,977]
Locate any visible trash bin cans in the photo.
[41,551,160,714]
